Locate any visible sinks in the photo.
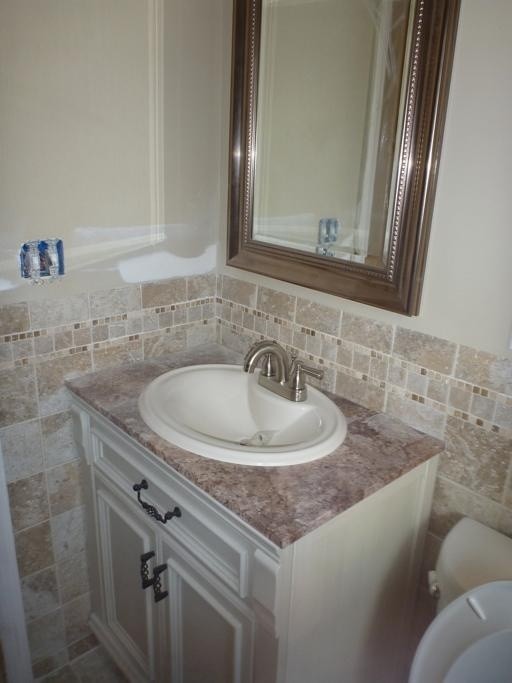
[136,363,348,467]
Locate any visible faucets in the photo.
[243,339,325,401]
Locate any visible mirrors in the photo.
[226,0,461,318]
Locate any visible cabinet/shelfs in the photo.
[70,390,441,683]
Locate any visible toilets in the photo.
[408,516,512,683]
